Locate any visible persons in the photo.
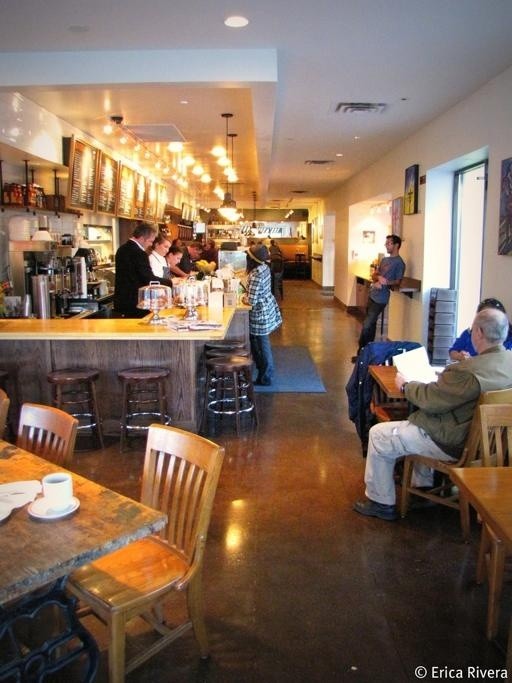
[351,234,406,362]
[165,244,184,269]
[448,298,512,360]
[147,231,172,280]
[241,244,283,386]
[268,239,282,261]
[353,307,512,521]
[170,242,205,277]
[112,221,179,318]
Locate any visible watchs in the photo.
[400,382,408,394]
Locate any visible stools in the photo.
[205,340,247,400]
[204,349,249,421]
[0,364,21,439]
[115,367,171,453]
[204,357,260,436]
[47,367,104,449]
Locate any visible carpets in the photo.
[252,346,328,393]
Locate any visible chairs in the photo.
[477,404,511,636]
[16,402,78,469]
[360,342,418,421]
[63,424,225,677]
[399,388,512,539]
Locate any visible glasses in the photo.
[384,242,394,245]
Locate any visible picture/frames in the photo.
[405,163,420,215]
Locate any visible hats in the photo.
[246,244,267,265]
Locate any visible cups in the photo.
[42,473,73,512]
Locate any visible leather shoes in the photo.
[355,500,397,520]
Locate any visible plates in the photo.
[28,497,80,519]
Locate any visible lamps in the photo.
[217,112,236,221]
[227,133,244,222]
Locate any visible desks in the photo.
[370,363,445,482]
[453,466,512,639]
[210,289,252,354]
[2,307,235,436]
[0,440,170,678]
